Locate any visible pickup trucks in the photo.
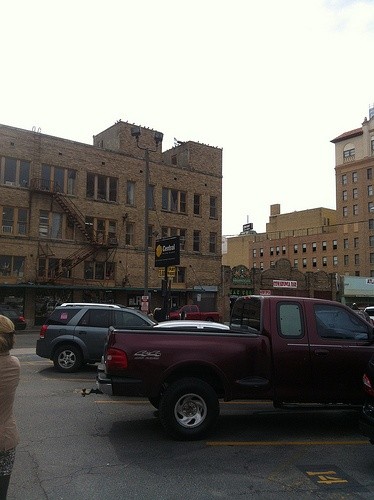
[96,294,374,441]
[167,304,220,324]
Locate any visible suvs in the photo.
[35,302,231,373]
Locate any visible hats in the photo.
[0,315,16,334]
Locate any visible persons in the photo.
[179,304,185,320]
[0,315,22,500]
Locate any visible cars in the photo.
[0,308,27,331]
[363,306,374,322]
